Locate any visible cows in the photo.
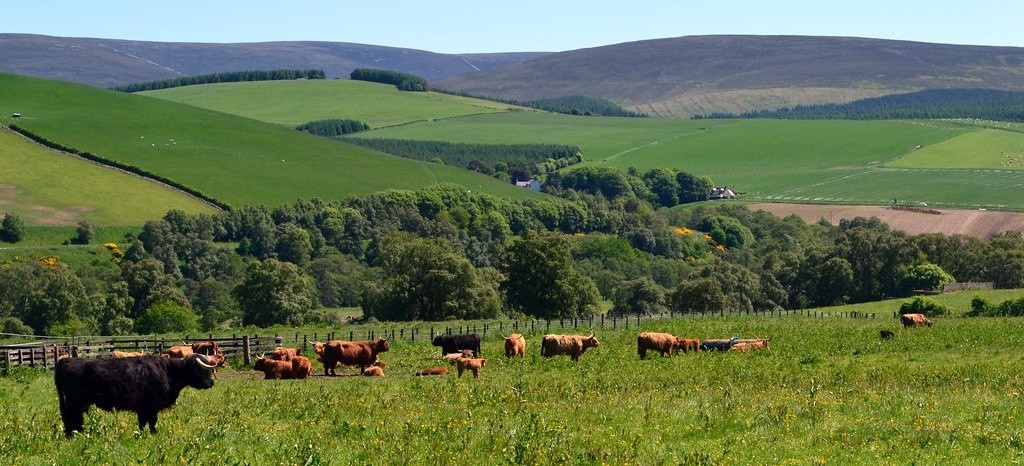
[38,341,229,441]
[416,331,488,379]
[900,314,933,329]
[541,329,600,363]
[500,332,526,358]
[253,333,391,379]
[638,331,774,359]
